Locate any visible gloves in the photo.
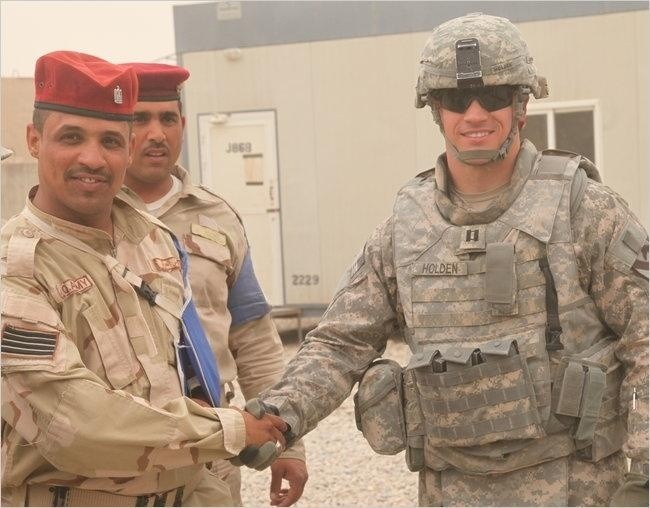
[226,397,294,472]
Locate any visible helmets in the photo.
[414,12,550,109]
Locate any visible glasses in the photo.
[434,85,514,113]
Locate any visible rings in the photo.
[275,439,282,456]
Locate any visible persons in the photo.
[222,12,649,508]
[116,63,308,507]
[1,50,289,507]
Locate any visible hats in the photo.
[116,62,190,101]
[34,50,139,122]
